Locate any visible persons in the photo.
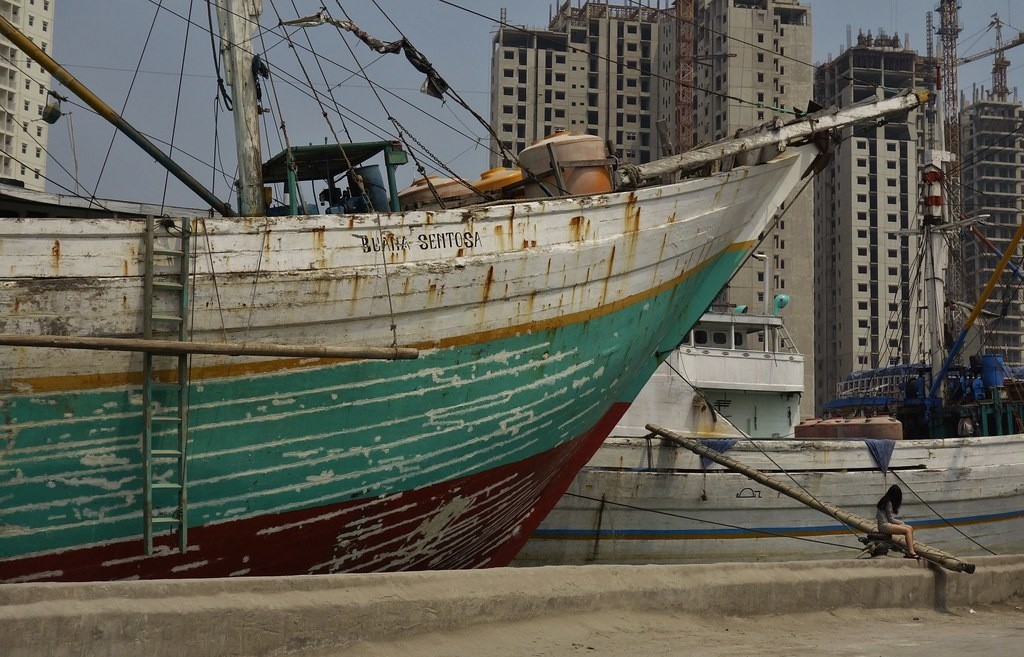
[875,483,922,559]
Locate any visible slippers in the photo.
[903,553,922,561]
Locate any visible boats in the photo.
[1,2,932,572]
[585,303,807,443]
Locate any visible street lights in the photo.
[751,252,772,352]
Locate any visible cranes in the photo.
[929,11,1024,103]
[933,0,965,311]
